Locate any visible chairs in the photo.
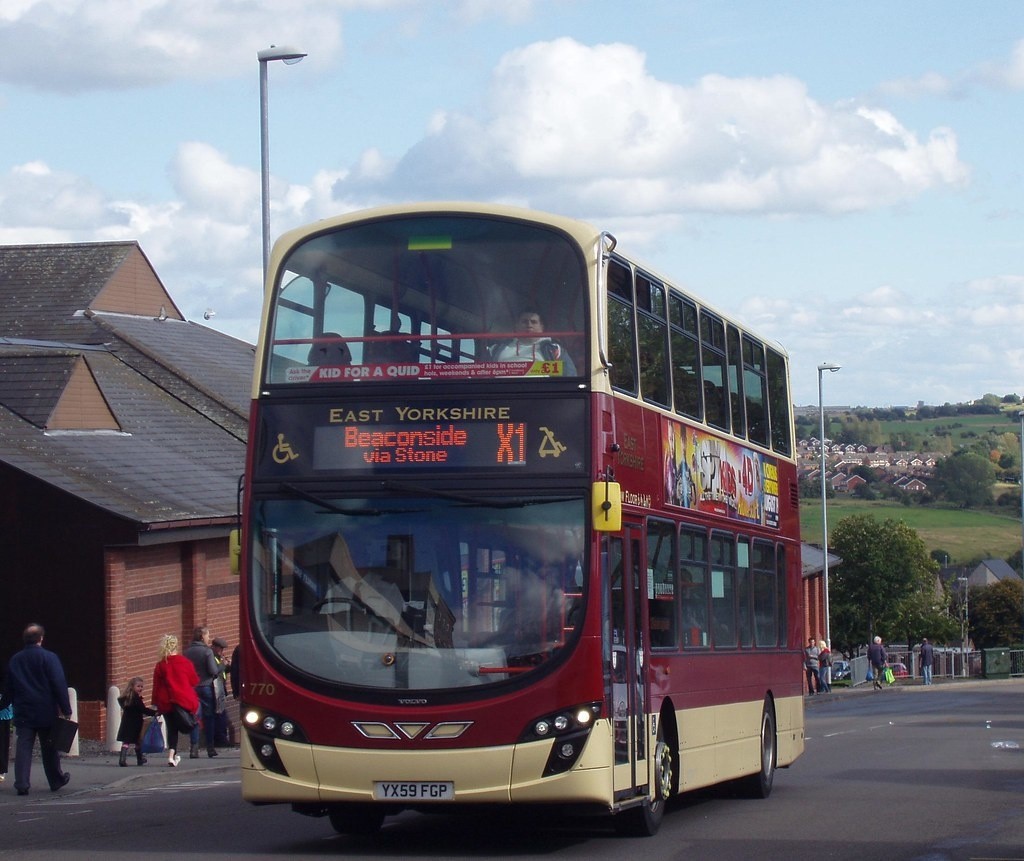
[672,368,767,444]
[366,331,420,368]
[306,332,352,367]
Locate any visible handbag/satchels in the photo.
[45,717,79,753]
[866,668,874,682]
[882,667,896,686]
[213,676,225,713]
[169,702,196,734]
[140,717,164,754]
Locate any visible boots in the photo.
[134,747,147,766]
[207,741,218,757]
[119,746,128,767]
[190,743,199,758]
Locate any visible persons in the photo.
[150,634,200,767]
[680,568,716,630]
[804,638,820,696]
[182,627,225,758]
[919,638,933,685]
[0,622,72,795]
[867,636,888,691]
[492,307,577,378]
[0,655,12,781]
[317,534,402,633]
[818,640,832,694]
[209,638,230,748]
[116,676,162,767]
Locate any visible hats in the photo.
[212,637,229,648]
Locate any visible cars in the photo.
[888,662,908,681]
[830,658,851,682]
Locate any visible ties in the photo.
[350,580,363,631]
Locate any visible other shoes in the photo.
[874,686,877,689]
[877,683,883,689]
[175,755,181,765]
[809,692,814,696]
[169,758,176,766]
[17,788,29,796]
[817,692,819,695]
[0,772,5,780]
[51,771,70,791]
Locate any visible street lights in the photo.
[816,360,842,685]
[253,43,309,293]
[956,576,970,664]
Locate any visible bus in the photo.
[225,202,808,839]
